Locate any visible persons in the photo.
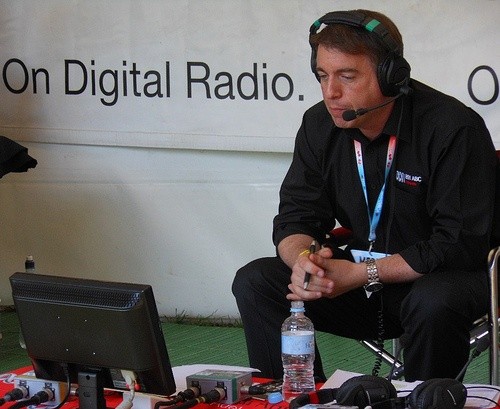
[231,9,500,381]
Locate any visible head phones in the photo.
[290,375,467,409]
[309,11,413,96]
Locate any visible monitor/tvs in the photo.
[9,272,177,409]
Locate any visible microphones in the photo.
[342,92,405,122]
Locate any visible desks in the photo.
[0,362,500,409]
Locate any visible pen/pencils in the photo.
[303,241,319,290]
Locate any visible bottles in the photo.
[19,256,37,350]
[280,301,315,403]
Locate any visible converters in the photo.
[186,369,252,405]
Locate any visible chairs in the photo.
[356,151,500,384]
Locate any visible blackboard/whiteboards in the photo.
[1,1,498,151]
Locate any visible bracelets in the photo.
[297,249,310,257]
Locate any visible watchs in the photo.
[363,258,384,293]
